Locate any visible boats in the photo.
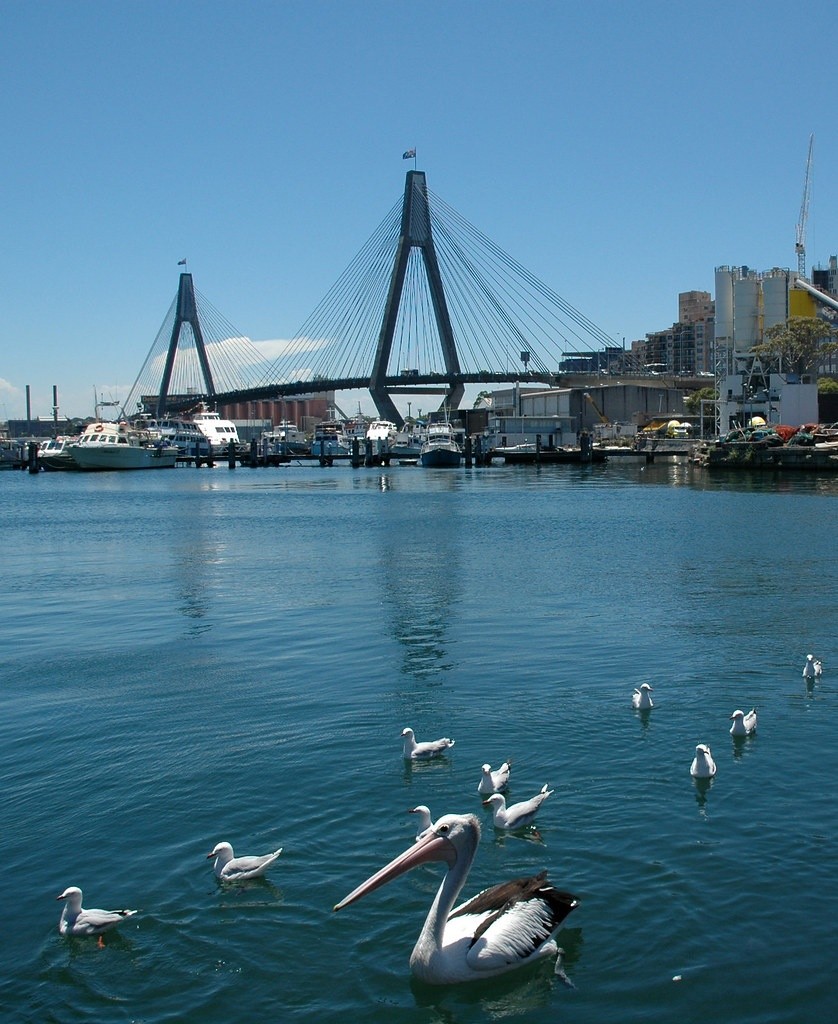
[495,443,536,452]
[310,409,350,455]
[419,423,463,464]
[255,422,309,453]
[132,410,240,456]
[0,428,77,469]
[350,401,426,456]
[65,402,177,469]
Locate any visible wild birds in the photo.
[631,682,655,709]
[801,654,823,678]
[56,885,140,935]
[206,840,283,881]
[728,708,758,736]
[400,727,455,759]
[690,743,717,778]
[333,760,581,986]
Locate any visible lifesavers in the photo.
[95,425,104,432]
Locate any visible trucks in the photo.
[666,425,690,439]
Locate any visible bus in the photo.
[400,369,419,377]
[641,363,669,376]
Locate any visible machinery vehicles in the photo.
[583,393,616,442]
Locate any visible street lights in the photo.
[618,333,626,374]
[679,330,691,374]
[598,349,604,374]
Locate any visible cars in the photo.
[695,371,714,378]
